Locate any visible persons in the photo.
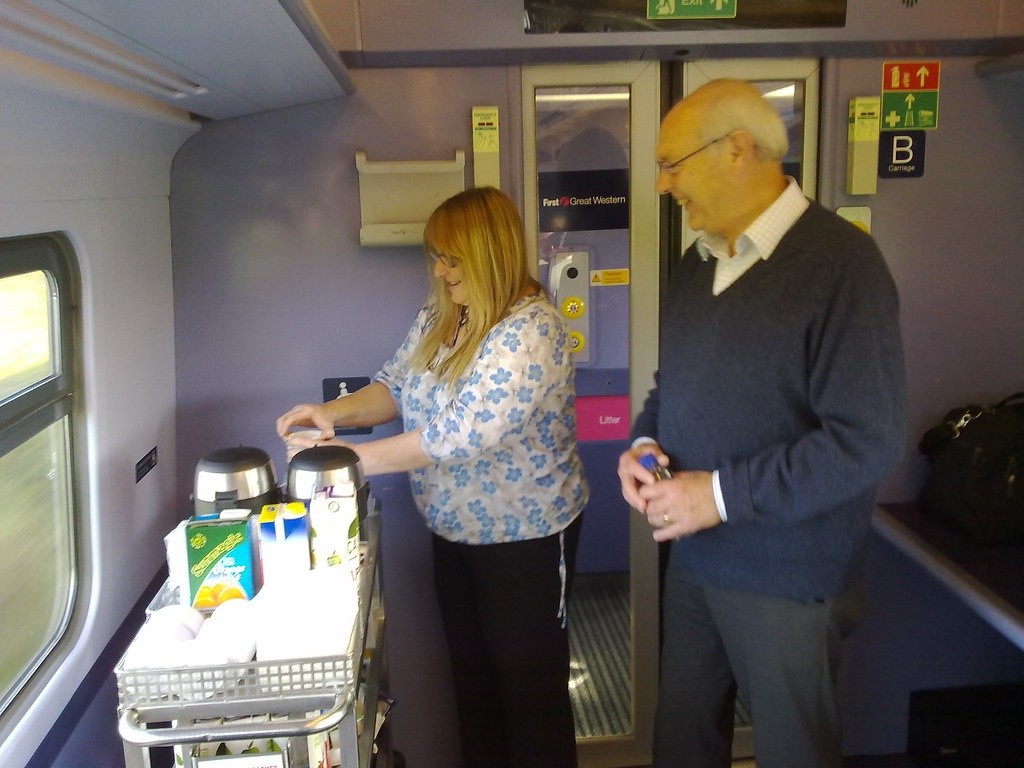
[616,76,905,768]
[276,186,592,768]
[539,128,628,368]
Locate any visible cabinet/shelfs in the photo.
[114,496,383,768]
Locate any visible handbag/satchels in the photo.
[915,393,1024,550]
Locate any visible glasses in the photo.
[656,132,758,176]
[428,250,463,268]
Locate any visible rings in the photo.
[663,512,672,526]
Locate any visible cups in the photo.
[124,598,255,698]
[285,430,326,462]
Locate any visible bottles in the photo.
[638,453,672,486]
